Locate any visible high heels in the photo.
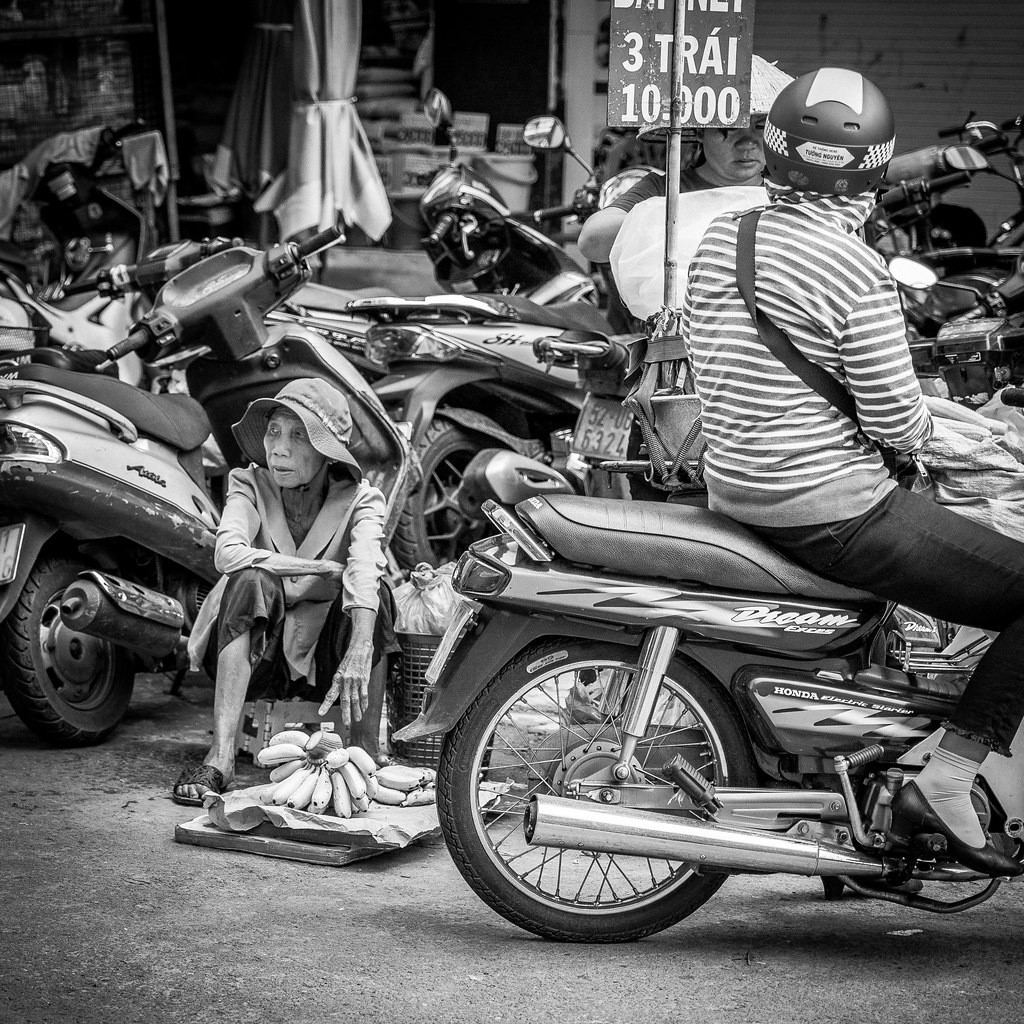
[890,779,1024,877]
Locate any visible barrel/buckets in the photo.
[472,152,539,214]
[383,185,432,251]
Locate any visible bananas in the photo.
[258,731,437,819]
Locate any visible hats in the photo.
[635,54,795,139]
[231,377,363,484]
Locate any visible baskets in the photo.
[386,634,447,770]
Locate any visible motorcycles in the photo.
[392,387,1023,943]
[1,87,1023,747]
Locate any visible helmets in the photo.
[762,67,896,196]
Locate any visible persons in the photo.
[684,69,1023,899]
[171,378,402,807]
[578,54,797,262]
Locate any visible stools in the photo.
[234,697,351,770]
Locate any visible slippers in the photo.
[172,765,228,806]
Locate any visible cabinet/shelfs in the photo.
[0,0,180,244]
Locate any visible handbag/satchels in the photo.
[852,431,933,494]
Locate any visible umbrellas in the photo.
[254,1,392,284]
